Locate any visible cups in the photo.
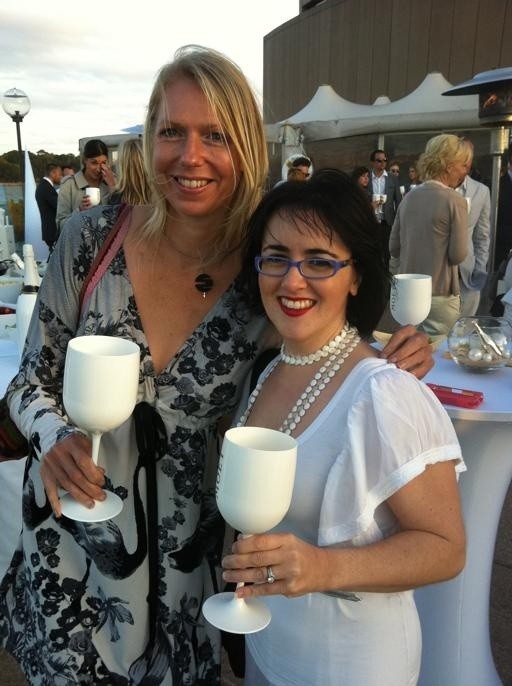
[390,273,433,325]
[86,187,100,208]
[410,184,416,191]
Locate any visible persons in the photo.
[34,139,150,263]
[0,44,436,686]
[286,168,306,181]
[218,167,469,686]
[291,156,310,171]
[352,133,512,338]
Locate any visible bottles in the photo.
[17,244,41,359]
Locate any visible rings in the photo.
[266,566,277,585]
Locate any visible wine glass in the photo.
[54,332,143,526]
[202,427,297,638]
[373,194,379,214]
[399,186,405,198]
[380,194,388,214]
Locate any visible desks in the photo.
[0,314,32,585]
[373,330,512,685]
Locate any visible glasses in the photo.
[255,256,352,278]
[376,158,388,163]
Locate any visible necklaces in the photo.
[163,229,215,299]
[235,325,362,437]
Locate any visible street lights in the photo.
[0,84,31,197]
